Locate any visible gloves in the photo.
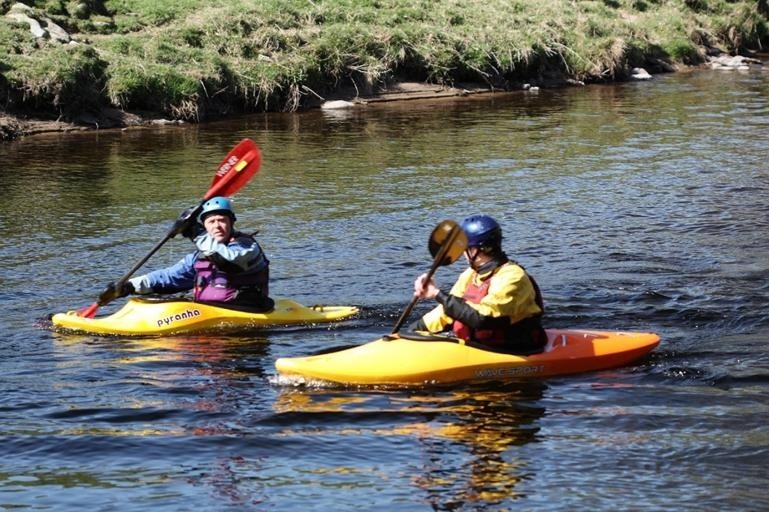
[174,210,206,242]
[97,280,135,306]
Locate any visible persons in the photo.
[401,214,550,353]
[96,196,272,308]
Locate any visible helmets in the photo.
[196,195,237,226]
[460,213,504,247]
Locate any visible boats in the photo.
[275,328,661,386]
[51,296,360,336]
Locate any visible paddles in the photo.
[390,219,468,333]
[76,138,262,320]
